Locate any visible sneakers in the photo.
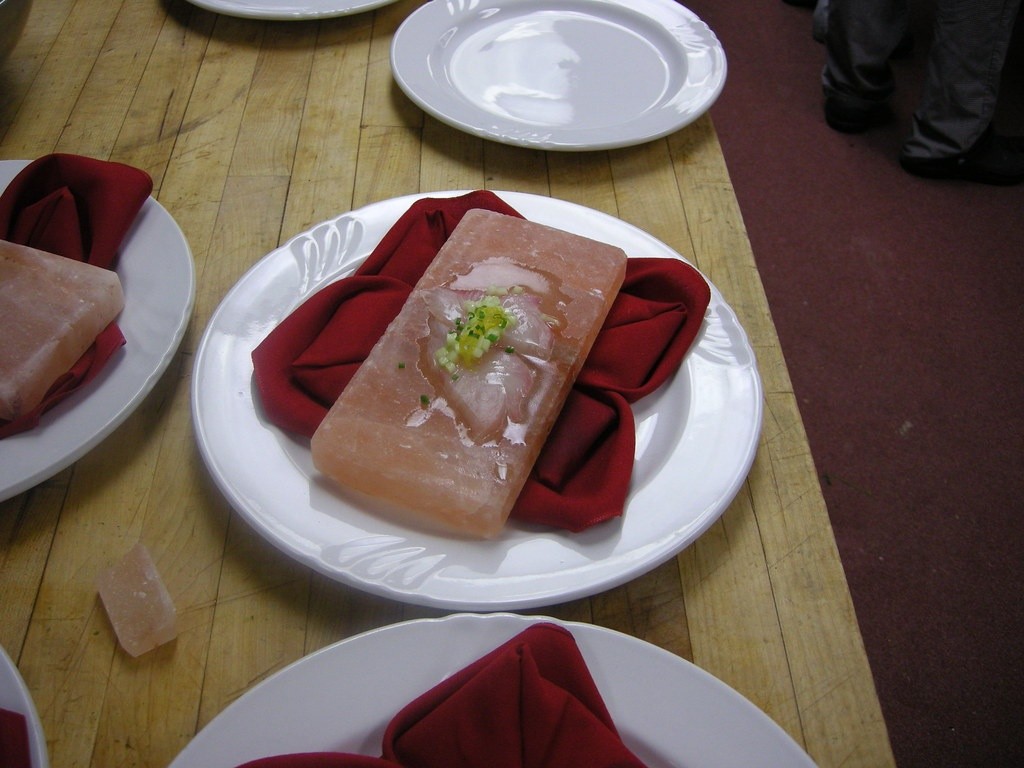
[825,92,873,134]
[899,123,1024,185]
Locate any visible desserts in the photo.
[93,544,177,658]
[0,240,124,419]
[310,209,627,537]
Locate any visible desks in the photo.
[0,0,897,768]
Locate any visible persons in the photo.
[821,0,1024,187]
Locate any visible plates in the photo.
[186,0,398,21]
[392,0,728,150]
[1,646,49,768]
[1,159,196,507]
[166,612,820,767]
[191,191,763,609]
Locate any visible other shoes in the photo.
[889,32,914,58]
